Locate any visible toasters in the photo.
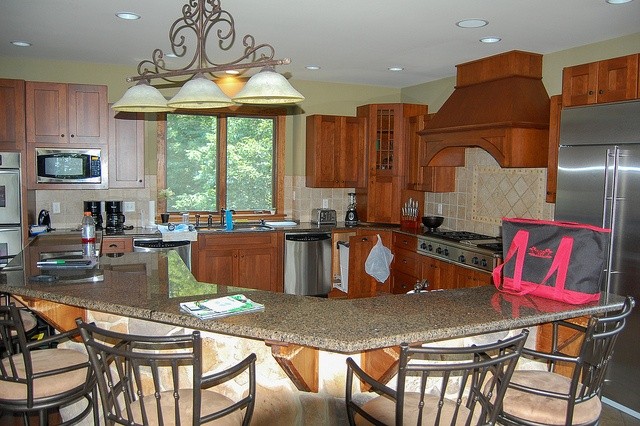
[312,209,337,225]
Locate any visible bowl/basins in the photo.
[422,217,443,230]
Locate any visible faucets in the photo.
[220,207,237,226]
[259,217,265,225]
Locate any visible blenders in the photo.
[344,193,358,226]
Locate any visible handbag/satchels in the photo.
[491,215,611,305]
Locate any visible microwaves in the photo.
[36,147,102,184]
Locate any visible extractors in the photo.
[419,129,549,168]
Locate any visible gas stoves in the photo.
[417,231,503,272]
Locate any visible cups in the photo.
[161,214,169,223]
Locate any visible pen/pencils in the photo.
[36,260,65,265]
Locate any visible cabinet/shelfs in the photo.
[192,232,282,291]
[357,102,427,226]
[0,79,28,259]
[26,83,109,189]
[332,230,392,298]
[546,95,563,202]
[306,114,366,187]
[108,101,146,189]
[391,232,417,293]
[562,54,640,106]
[421,256,493,290]
[407,113,456,191]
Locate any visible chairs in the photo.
[2,293,52,354]
[498,295,636,426]
[0,303,99,426]
[75,316,256,426]
[346,328,529,426]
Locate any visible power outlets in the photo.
[124,201,136,214]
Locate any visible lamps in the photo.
[111,0,305,114]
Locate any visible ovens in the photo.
[1,153,23,270]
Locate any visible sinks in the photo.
[237,224,273,231]
[195,225,232,232]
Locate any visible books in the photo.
[180,292,265,322]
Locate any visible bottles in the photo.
[81,212,96,257]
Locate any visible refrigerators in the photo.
[554,100,640,419]
